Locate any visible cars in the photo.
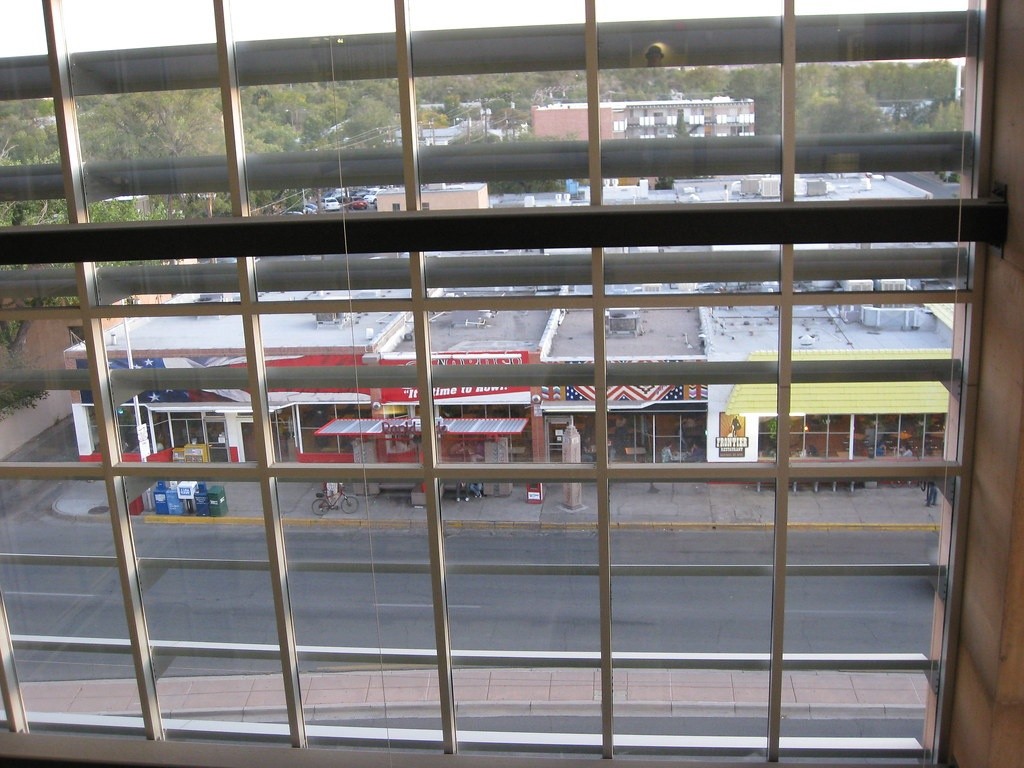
[286,185,398,215]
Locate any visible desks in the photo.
[891,433,912,440]
[885,449,902,456]
[836,451,849,458]
[847,433,865,440]
[671,451,689,462]
[625,446,646,456]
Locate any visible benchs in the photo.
[378,482,415,489]
[443,483,483,491]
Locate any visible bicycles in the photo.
[311,483,359,516]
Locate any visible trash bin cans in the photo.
[139,481,229,521]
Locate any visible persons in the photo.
[758,439,937,506]
[456,481,482,501]
[326,482,344,509]
[582,436,702,464]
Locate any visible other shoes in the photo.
[456,497,461,502]
[465,497,469,501]
[475,495,477,497]
[479,495,481,497]
